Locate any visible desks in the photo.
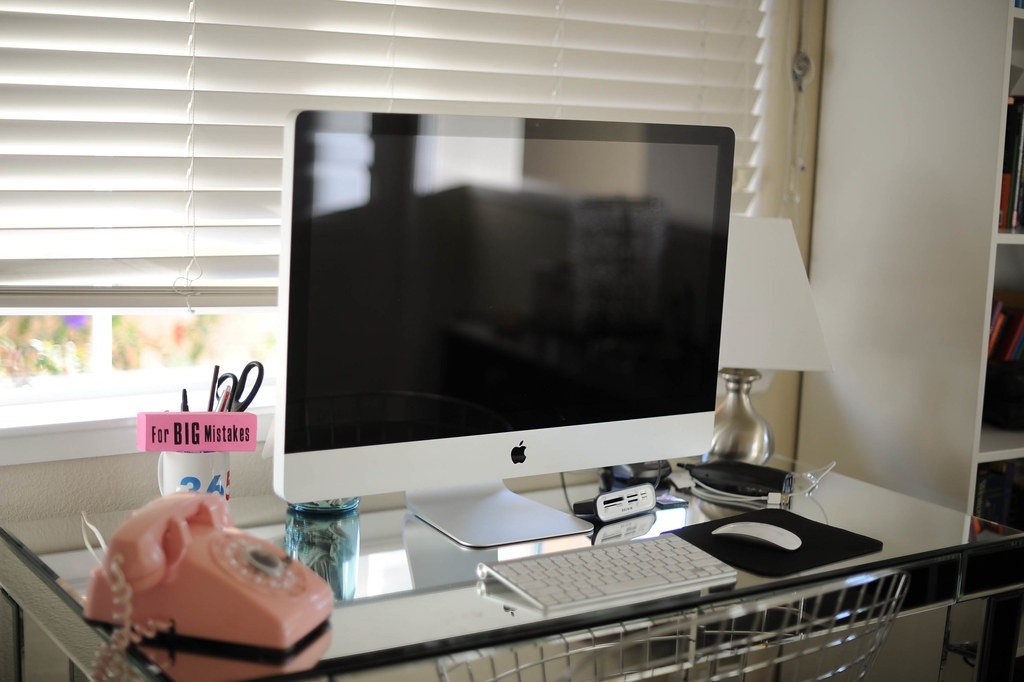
[0,452,1024,682]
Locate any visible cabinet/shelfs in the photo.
[776,0,1024,681]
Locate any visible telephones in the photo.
[84,490,335,682]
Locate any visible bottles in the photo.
[281,498,360,602]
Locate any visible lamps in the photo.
[701,212,831,466]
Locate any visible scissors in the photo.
[213,360,264,414]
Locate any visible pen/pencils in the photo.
[180,364,234,414]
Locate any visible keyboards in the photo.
[478,532,738,611]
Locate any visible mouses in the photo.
[712,522,802,550]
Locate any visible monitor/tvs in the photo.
[274,107,735,548]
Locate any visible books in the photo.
[998,95,1024,234]
[988,287,1024,431]
[975,460,1024,526]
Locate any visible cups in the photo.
[156,451,232,510]
